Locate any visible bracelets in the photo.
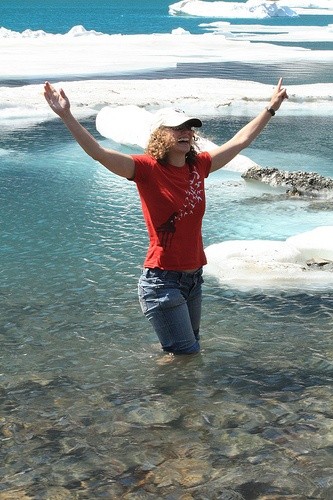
[265,105,276,117]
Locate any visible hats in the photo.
[156,106,202,128]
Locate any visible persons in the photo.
[43,77,289,356]
